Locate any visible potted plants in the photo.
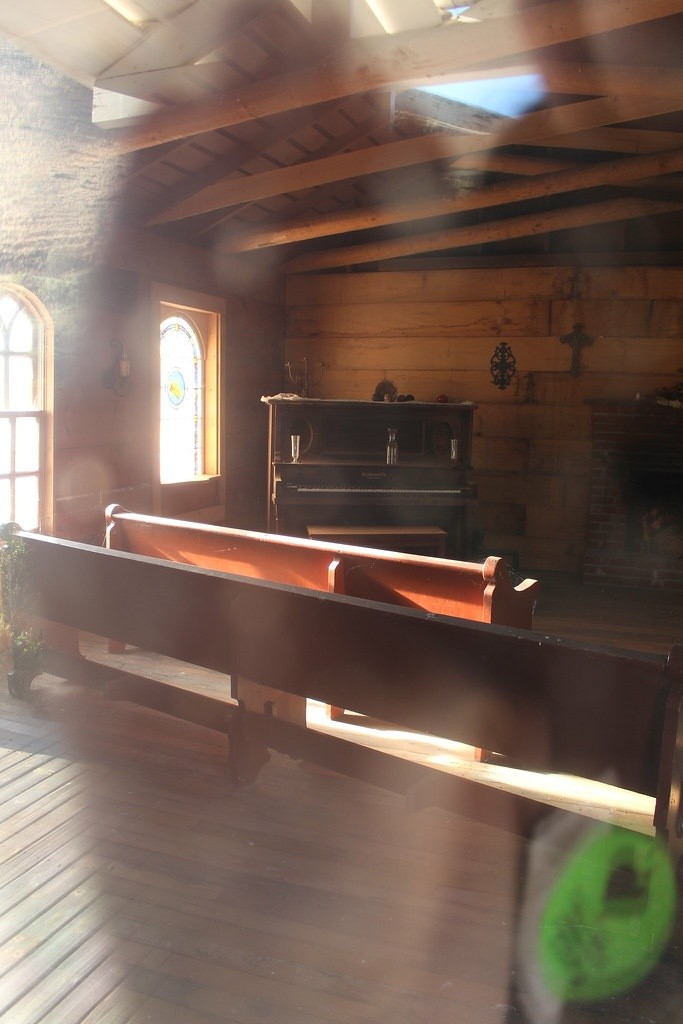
[0,526,51,700]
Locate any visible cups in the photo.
[450,438,458,461]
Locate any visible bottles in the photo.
[386,425,399,464]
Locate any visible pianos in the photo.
[259,391,481,562]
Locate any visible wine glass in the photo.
[291,435,300,464]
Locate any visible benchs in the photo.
[104,502,541,767]
[305,522,448,561]
